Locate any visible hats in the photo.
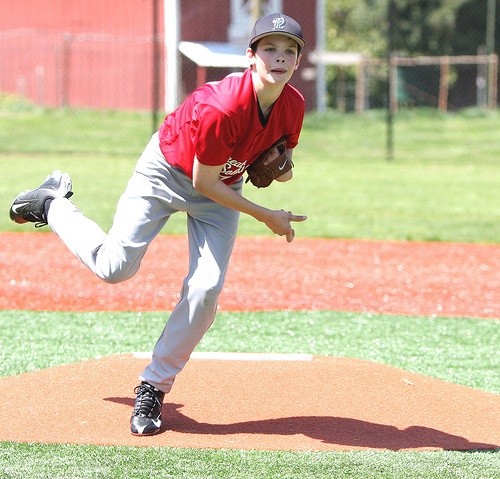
[248,12,306,53]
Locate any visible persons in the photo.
[10,13,306,433]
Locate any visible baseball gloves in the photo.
[245,138,294,189]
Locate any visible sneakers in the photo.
[9,170,74,228]
[130,381,164,436]
